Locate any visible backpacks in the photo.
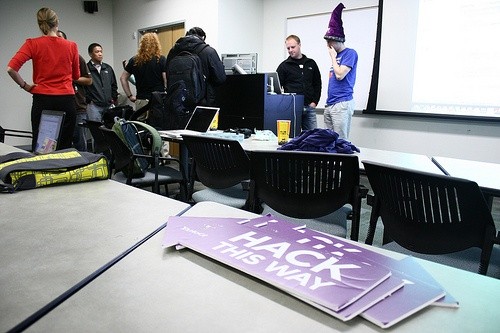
[166,42,207,111]
[111,116,162,170]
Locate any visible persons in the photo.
[276,35,323,133]
[7,6,93,151]
[85,42,119,127]
[120,30,168,115]
[323,4,359,143]
[164,26,228,120]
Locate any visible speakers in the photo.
[84,1,98,13]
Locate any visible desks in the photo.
[157,130,500,245]
[0,141,500,333]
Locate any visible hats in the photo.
[323,2,346,42]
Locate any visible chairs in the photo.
[252,150,368,242]
[98,125,185,200]
[78,119,114,179]
[361,160,500,276]
[180,134,256,212]
[120,123,150,168]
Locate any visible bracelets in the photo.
[20,81,26,89]
[128,94,133,98]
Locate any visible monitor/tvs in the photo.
[221,53,258,75]
[32,109,66,155]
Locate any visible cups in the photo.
[210,111,219,131]
[277,120,291,146]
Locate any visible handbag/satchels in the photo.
[0,149,109,192]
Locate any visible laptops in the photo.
[263,72,297,95]
[159,106,221,135]
